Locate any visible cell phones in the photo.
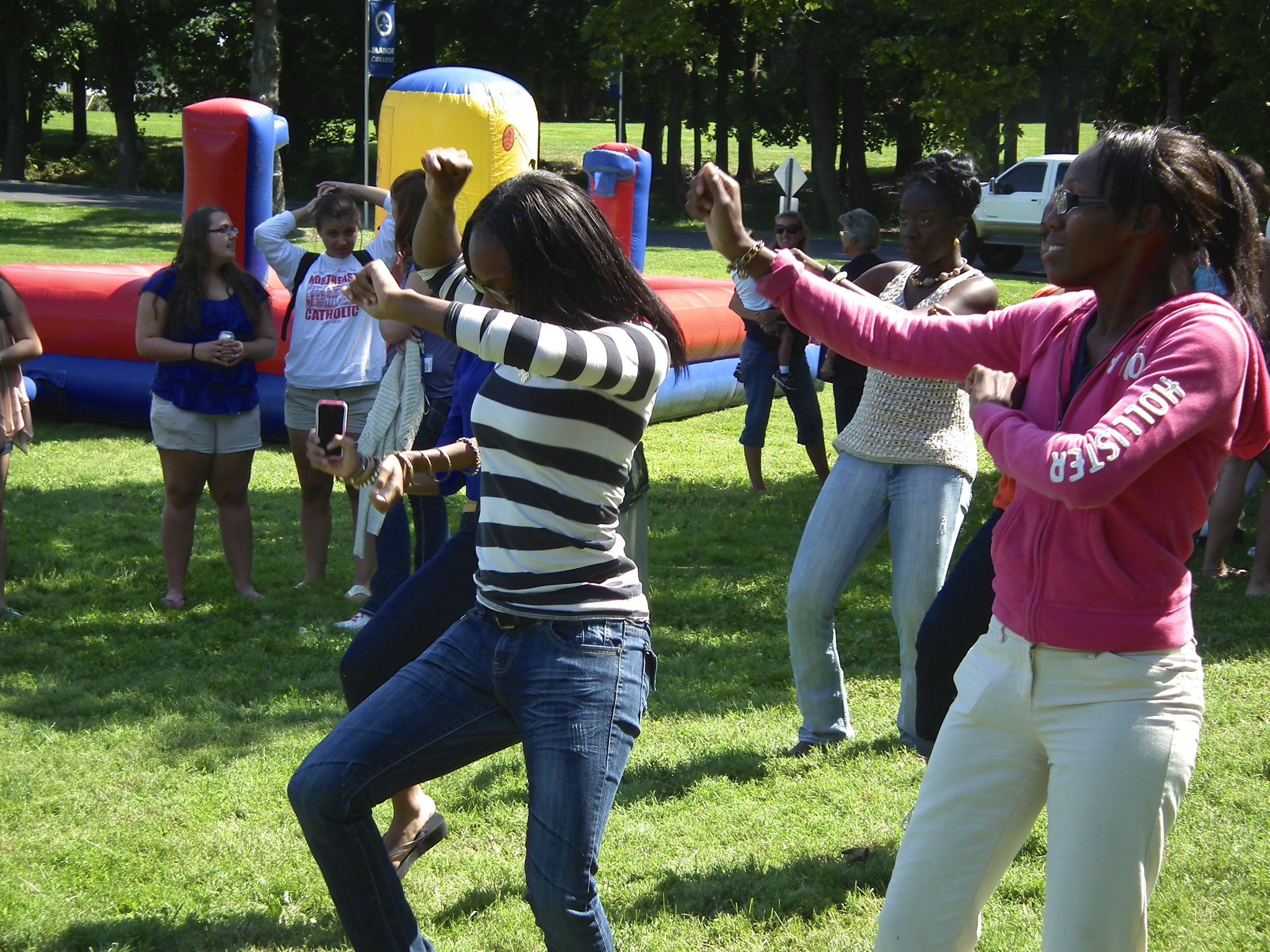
[315,399,350,463]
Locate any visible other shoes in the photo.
[783,738,823,755]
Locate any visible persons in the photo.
[134,206,278,605]
[687,124,1270,952]
[255,181,400,602]
[286,148,688,952]
[0,273,44,621]
[817,207,882,435]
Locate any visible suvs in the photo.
[960,154,1079,273]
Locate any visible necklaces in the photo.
[911,257,969,285]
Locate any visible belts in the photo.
[485,608,546,630]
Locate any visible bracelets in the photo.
[413,450,432,487]
[192,343,198,360]
[429,447,451,482]
[724,239,766,279]
[337,454,371,486]
[383,450,415,491]
[352,456,382,490]
[456,436,481,476]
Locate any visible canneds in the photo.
[218,331,235,363]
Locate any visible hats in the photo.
[838,209,882,250]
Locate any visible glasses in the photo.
[208,227,239,234]
[839,230,851,239]
[774,224,800,234]
[1053,189,1119,216]
[461,269,518,306]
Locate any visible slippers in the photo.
[162,596,186,607]
[378,813,447,879]
[4,607,22,620]
[246,594,264,600]
[292,580,316,593]
[343,585,373,601]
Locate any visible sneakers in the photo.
[771,369,800,390]
[333,612,371,628]
[733,363,744,383]
